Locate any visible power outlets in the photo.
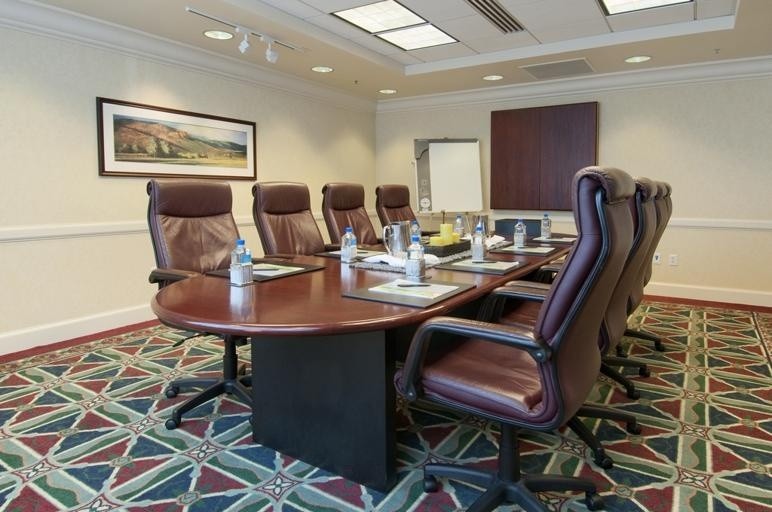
[668,254,679,265]
[652,253,663,265]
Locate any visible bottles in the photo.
[406,236,425,278]
[540,214,552,239]
[410,221,421,245]
[453,215,464,237]
[229,285,254,325]
[340,228,358,262]
[230,240,254,285]
[513,218,527,248]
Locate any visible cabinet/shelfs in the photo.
[490,101,599,211]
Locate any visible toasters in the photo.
[472,225,486,260]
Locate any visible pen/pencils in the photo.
[357,252,368,253]
[465,212,472,233]
[442,210,445,223]
[398,285,431,287]
[253,269,279,271]
[472,261,497,263]
[518,246,537,248]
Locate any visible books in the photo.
[527,236,577,244]
[316,249,387,261]
[436,257,530,277]
[488,245,561,257]
[339,278,476,309]
[206,261,327,283]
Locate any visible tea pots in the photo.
[382,221,409,256]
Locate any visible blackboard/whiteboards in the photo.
[414,137,483,214]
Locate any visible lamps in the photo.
[238,33,252,56]
[264,43,278,63]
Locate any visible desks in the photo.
[150,233,578,492]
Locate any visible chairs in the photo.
[252,181,341,260]
[616,182,672,357]
[321,182,388,246]
[551,182,671,401]
[375,184,439,236]
[505,176,657,467]
[146,178,284,429]
[394,165,635,512]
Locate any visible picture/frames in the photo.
[96,96,257,181]
